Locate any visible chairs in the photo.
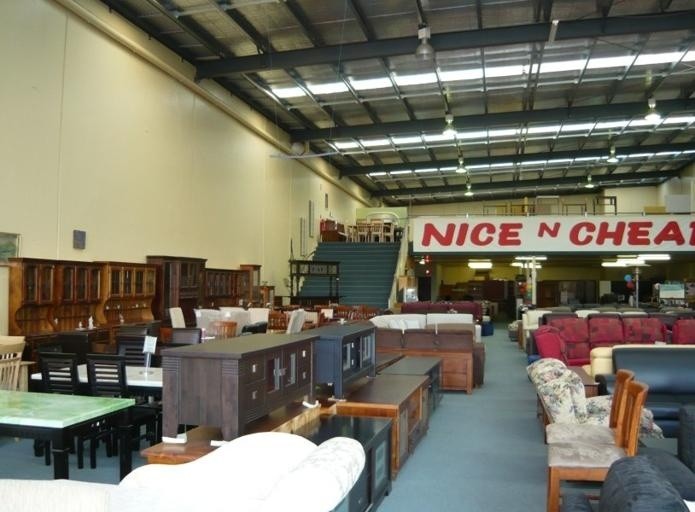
[170,328,202,345]
[96,338,152,457]
[0,335,27,359]
[383,222,395,243]
[0,342,29,439]
[133,342,189,451]
[285,307,307,333]
[546,367,637,495]
[548,380,649,512]
[209,320,239,341]
[167,306,186,327]
[84,352,156,471]
[337,305,355,318]
[370,220,384,243]
[269,313,289,329]
[247,307,270,325]
[192,308,218,329]
[38,351,85,468]
[356,219,370,243]
[219,307,244,323]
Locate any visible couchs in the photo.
[428,303,456,314]
[369,313,426,331]
[623,317,668,345]
[519,310,551,351]
[529,312,579,356]
[400,302,429,313]
[562,455,693,512]
[676,401,695,471]
[672,318,695,344]
[588,318,625,352]
[532,325,570,365]
[2,430,367,511]
[537,302,695,323]
[426,312,482,343]
[549,317,590,366]
[524,356,662,444]
[595,345,695,436]
[371,327,476,395]
[452,302,478,315]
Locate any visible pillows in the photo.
[403,318,421,330]
[387,318,407,331]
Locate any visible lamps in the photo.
[416,25,438,68]
[443,114,456,139]
[644,97,663,121]
[456,157,467,175]
[605,145,619,163]
[463,183,475,197]
[585,173,594,189]
[291,143,305,155]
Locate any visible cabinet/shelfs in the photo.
[94,260,163,347]
[8,256,108,384]
[438,279,613,307]
[240,264,260,308]
[204,268,250,309]
[260,285,275,309]
[286,259,346,306]
[146,255,208,328]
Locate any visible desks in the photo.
[159,332,321,441]
[303,318,379,401]
[139,402,336,464]
[291,412,395,511]
[378,352,445,419]
[0,359,38,392]
[346,224,391,243]
[374,351,405,373]
[0,389,140,483]
[335,373,432,483]
